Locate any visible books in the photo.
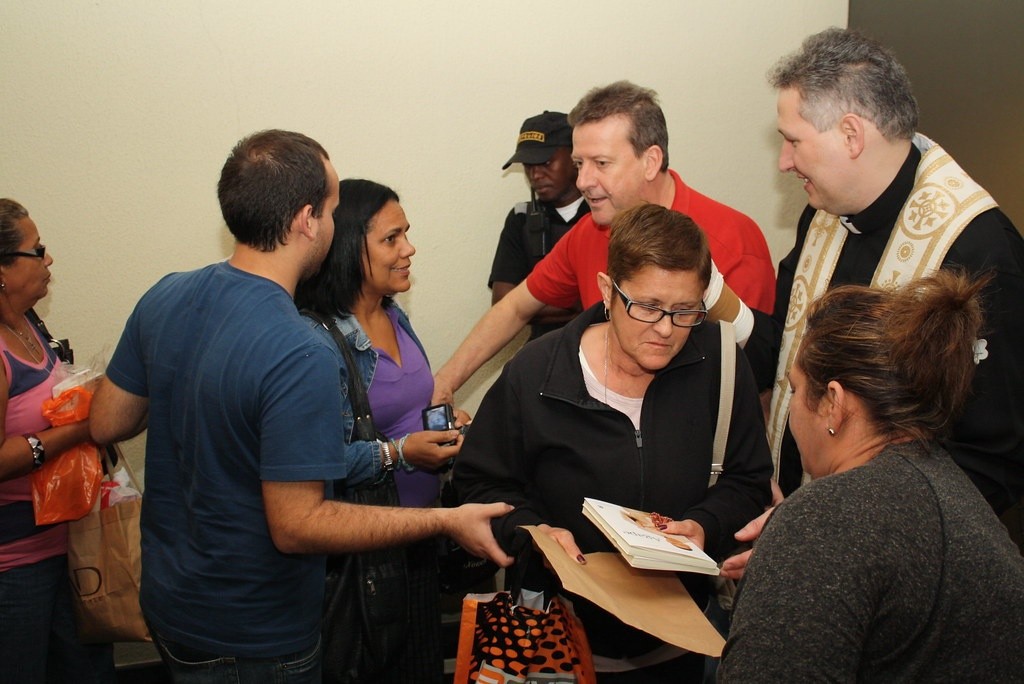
[579,497,720,578]
[51,369,103,412]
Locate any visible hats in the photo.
[502,110,574,170]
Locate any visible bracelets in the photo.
[23,433,45,469]
[374,433,414,484]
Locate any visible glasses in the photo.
[608,272,708,328]
[0,246,45,263]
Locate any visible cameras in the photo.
[422,404,460,447]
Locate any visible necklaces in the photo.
[605,324,611,406]
[4,323,59,379]
[6,323,42,357]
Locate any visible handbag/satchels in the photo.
[29,388,104,526]
[454,538,596,684]
[70,439,153,643]
[317,430,445,684]
[28,306,74,365]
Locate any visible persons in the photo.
[716,271,1024,683]
[487,112,592,348]
[720,28,1024,578]
[89,129,515,684]
[622,507,693,551]
[292,179,472,684]
[431,80,777,410]
[0,199,120,684]
[443,204,776,684]
